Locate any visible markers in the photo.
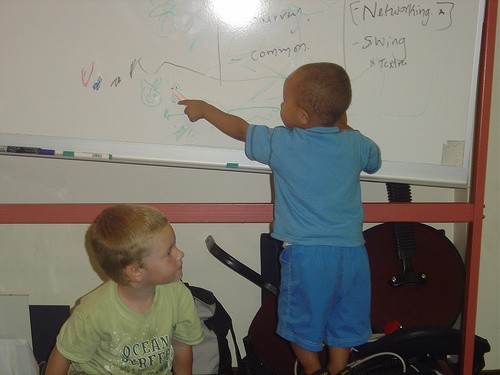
[0,145,41,154]
[40,149,64,156]
[171,88,189,108]
[63,151,113,160]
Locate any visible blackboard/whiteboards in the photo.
[0,0,488,190]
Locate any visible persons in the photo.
[44,205,204,375]
[176,62,382,374]
